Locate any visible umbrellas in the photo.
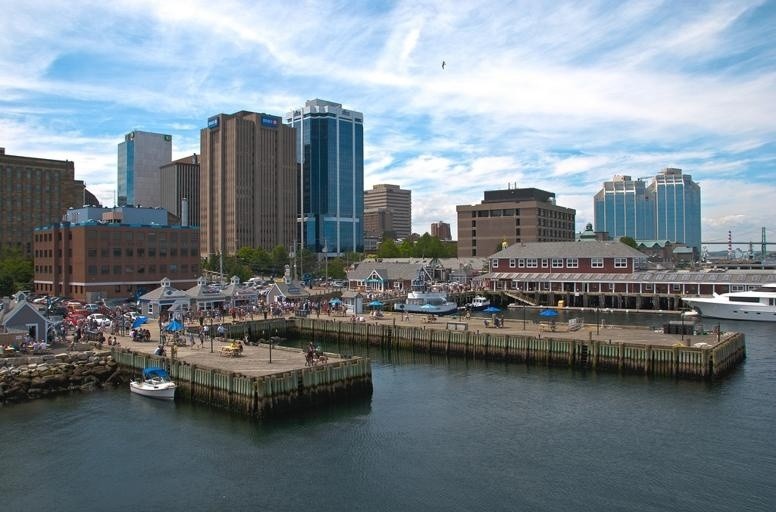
[368,300,384,307]
[539,308,560,323]
[484,305,504,313]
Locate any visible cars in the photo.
[123,311,148,324]
[86,313,110,326]
[33,295,99,314]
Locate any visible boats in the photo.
[130,367,178,401]
[680,282,776,324]
[468,297,490,310]
[393,290,457,314]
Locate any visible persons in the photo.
[370,307,385,317]
[550,319,556,332]
[499,315,504,327]
[404,309,410,321]
